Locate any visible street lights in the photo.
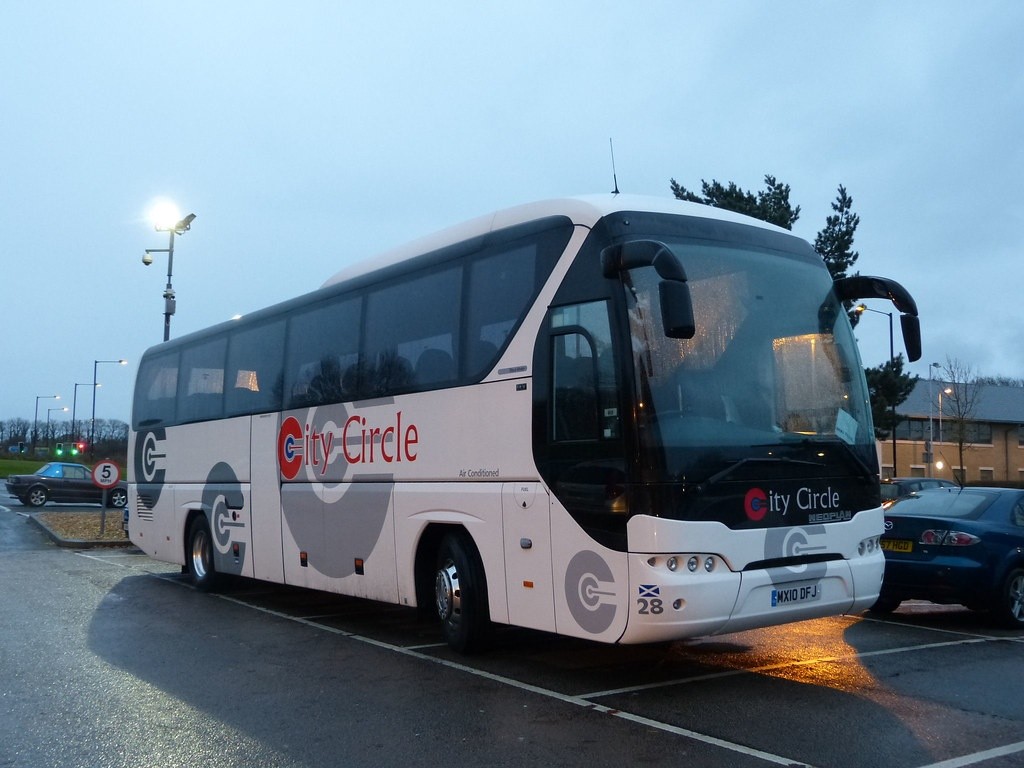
[139,198,195,400]
[939,386,952,478]
[928,362,941,478]
[46,407,68,448]
[32,395,61,455]
[857,303,898,478]
[71,383,103,443]
[90,359,129,464]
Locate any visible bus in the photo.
[126,187,926,657]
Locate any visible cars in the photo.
[4,461,128,509]
[881,477,960,504]
[867,483,1024,633]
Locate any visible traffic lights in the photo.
[18,442,25,455]
[55,442,64,456]
[77,443,84,455]
[71,443,78,455]
